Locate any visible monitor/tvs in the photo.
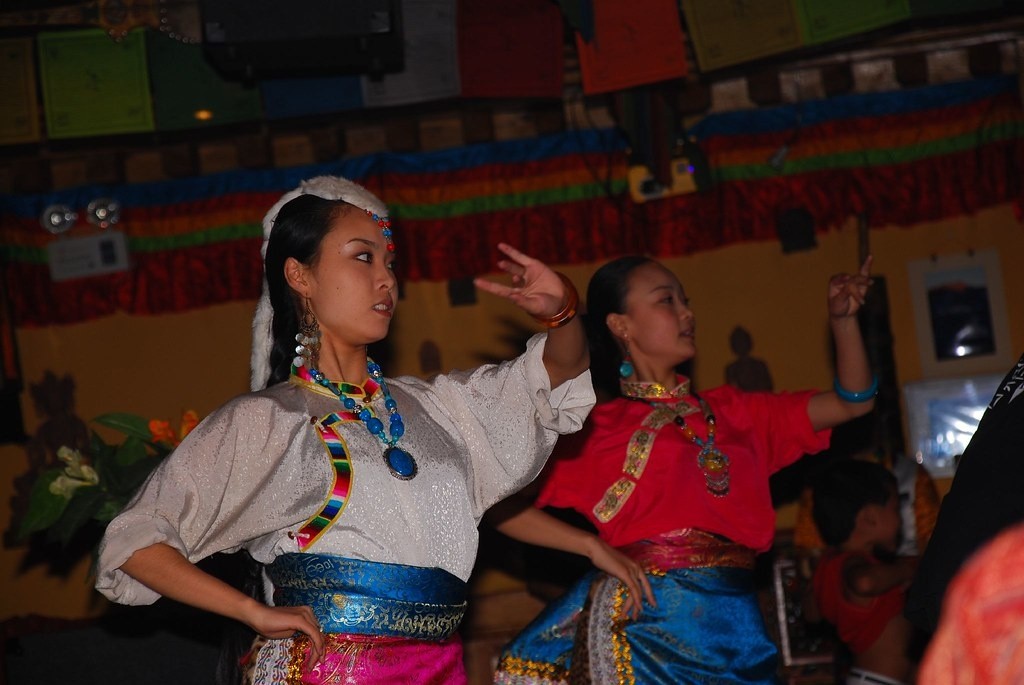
[200,0,406,75]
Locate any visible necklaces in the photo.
[623,391,731,497]
[308,361,417,481]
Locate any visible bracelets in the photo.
[540,289,580,328]
[527,271,575,321]
[834,369,879,403]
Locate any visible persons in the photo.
[94,175,596,685]
[902,351,1024,685]
[486,254,881,685]
[492,556,596,685]
[810,459,913,685]
[794,415,939,623]
